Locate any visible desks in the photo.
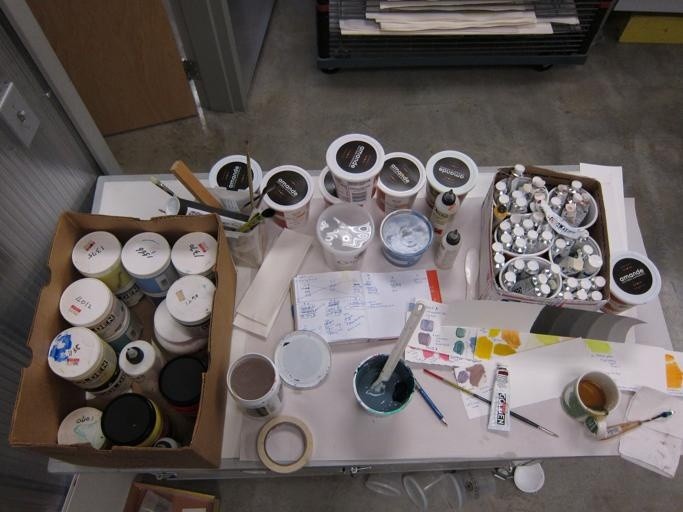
[47,163,683,474]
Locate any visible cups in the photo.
[349,352,417,417]
[224,351,285,423]
[559,370,623,425]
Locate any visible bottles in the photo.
[362,471,497,511]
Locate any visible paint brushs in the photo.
[414,369,558,438]
[148,138,279,231]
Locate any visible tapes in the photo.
[256,415,312,474]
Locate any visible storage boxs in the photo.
[9,210,237,469]
[122,481,220,512]
[615,12,683,45]
[478,166,612,311]
